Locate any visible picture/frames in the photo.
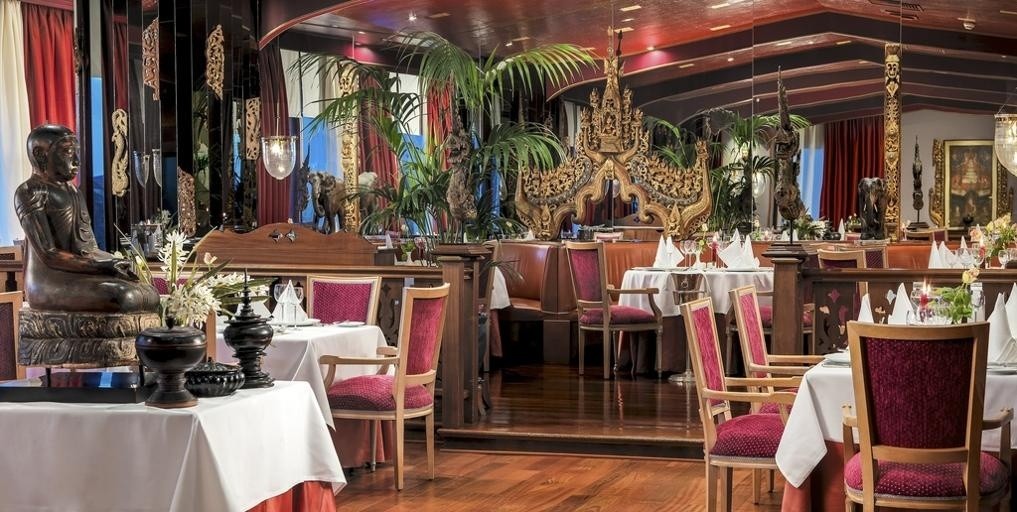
[943,138,998,231]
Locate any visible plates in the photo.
[283,318,321,326]
[630,266,773,272]
[335,321,366,327]
[824,353,850,364]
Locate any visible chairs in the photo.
[303,273,451,492]
[564,241,664,380]
[817,244,890,272]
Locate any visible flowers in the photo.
[111,220,278,322]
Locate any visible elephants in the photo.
[308,171,345,234]
[858,176,890,240]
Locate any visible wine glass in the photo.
[945,248,1017,271]
[679,237,728,274]
[273,283,304,331]
[906,288,986,326]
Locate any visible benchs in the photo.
[493,239,775,367]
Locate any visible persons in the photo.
[14,124,158,313]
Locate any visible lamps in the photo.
[993,84,1017,178]
[260,35,299,181]
